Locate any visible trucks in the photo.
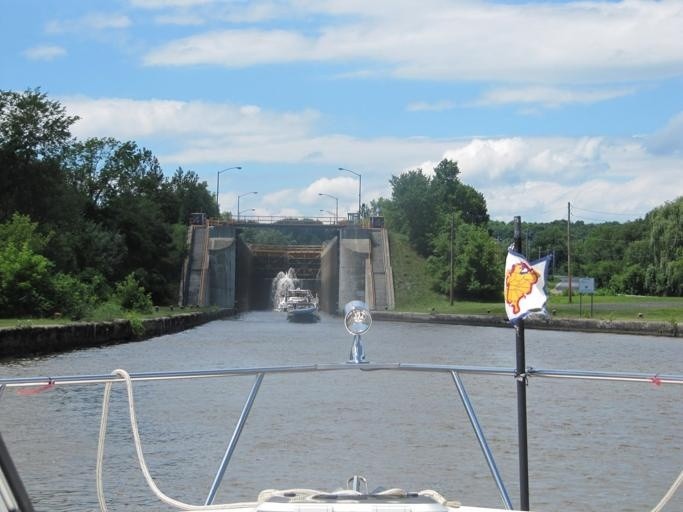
[556,277,595,292]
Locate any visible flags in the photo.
[504,243,553,323]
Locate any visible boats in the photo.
[275,288,319,312]
[287,301,317,317]
[1,286,682,512]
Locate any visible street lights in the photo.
[215,166,242,206]
[237,191,257,221]
[318,194,339,224]
[338,167,362,213]
